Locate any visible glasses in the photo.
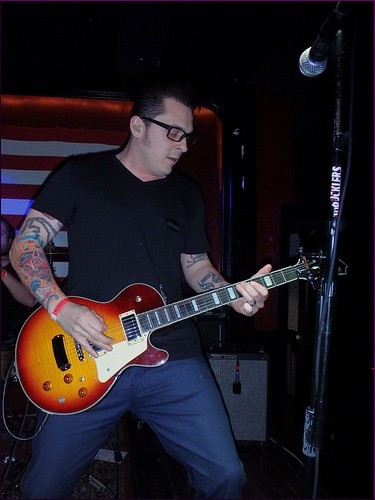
[142,116,196,149]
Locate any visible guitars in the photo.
[14,246,348,416]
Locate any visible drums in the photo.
[0,342,18,387]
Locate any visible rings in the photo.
[251,299,257,306]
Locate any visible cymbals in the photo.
[45,250,68,255]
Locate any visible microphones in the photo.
[233,354,242,395]
[298,0,349,77]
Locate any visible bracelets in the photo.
[50,297,71,322]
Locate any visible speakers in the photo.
[206,354,270,443]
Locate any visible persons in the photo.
[0,218,40,430]
[8,80,273,500]
